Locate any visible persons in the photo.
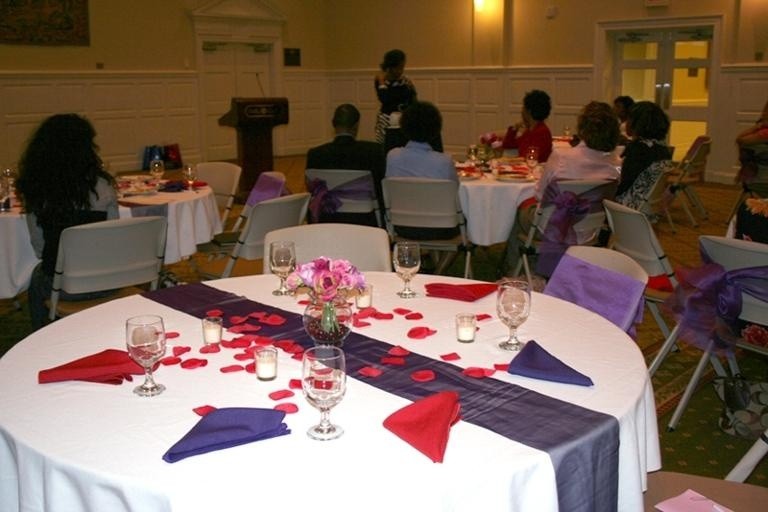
[613,95,636,148]
[15,113,119,331]
[373,49,417,148]
[304,103,385,228]
[486,102,622,283]
[503,89,552,163]
[386,101,461,267]
[593,101,673,248]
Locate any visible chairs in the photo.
[642,470,768,511]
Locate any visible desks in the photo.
[1,273,662,511]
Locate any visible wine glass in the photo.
[497,281,532,351]
[182,165,198,194]
[527,146,538,181]
[151,160,165,188]
[269,241,296,296]
[301,345,346,442]
[126,315,167,397]
[392,242,422,299]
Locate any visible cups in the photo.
[253,346,278,381]
[202,316,223,346]
[356,283,373,310]
[456,313,477,343]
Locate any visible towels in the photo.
[162,408,292,464]
[382,389,461,462]
